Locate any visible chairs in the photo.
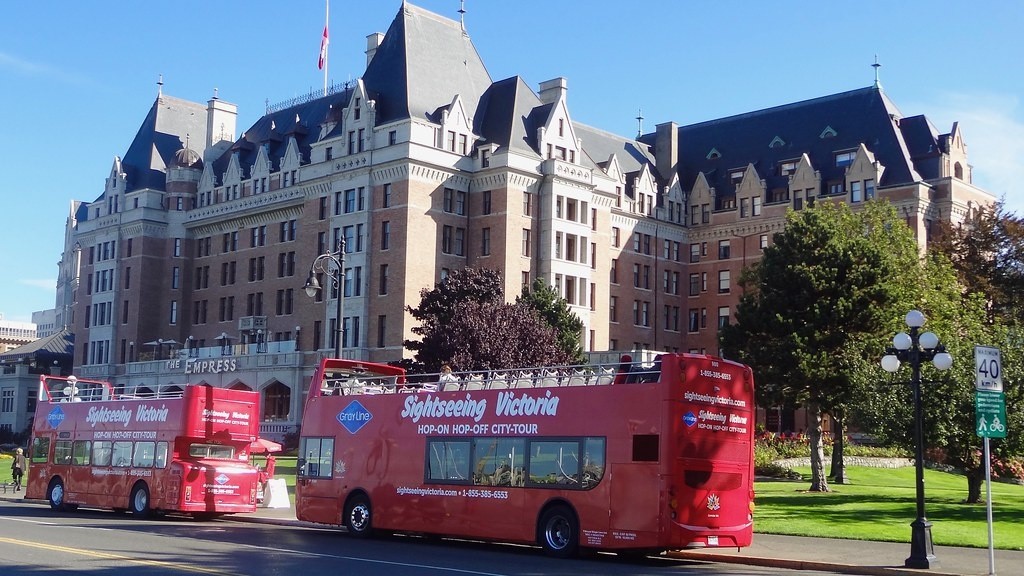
[443,376,462,391]
[567,368,587,386]
[542,369,559,386]
[509,375,518,388]
[516,371,534,388]
[423,384,439,392]
[490,372,508,389]
[439,373,447,391]
[588,371,602,385]
[466,373,484,390]
[462,376,469,390]
[418,388,436,393]
[560,371,570,386]
[596,367,616,386]
[398,389,416,393]
[485,377,492,389]
[535,373,545,387]
[321,378,395,396]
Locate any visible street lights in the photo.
[300,233,351,361]
[881,307,953,569]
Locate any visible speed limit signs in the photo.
[975,347,1002,391]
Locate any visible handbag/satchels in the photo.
[10,454,20,470]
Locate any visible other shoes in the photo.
[17,487,21,491]
[13,483,19,488]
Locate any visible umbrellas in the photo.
[250,439,282,466]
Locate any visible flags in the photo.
[318,26,328,69]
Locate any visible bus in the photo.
[23,376,260,515]
[287,358,755,564]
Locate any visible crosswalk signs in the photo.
[974,392,1007,438]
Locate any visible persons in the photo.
[257,453,276,502]
[437,365,457,391]
[613,355,632,384]
[11,448,26,493]
[641,355,662,384]
